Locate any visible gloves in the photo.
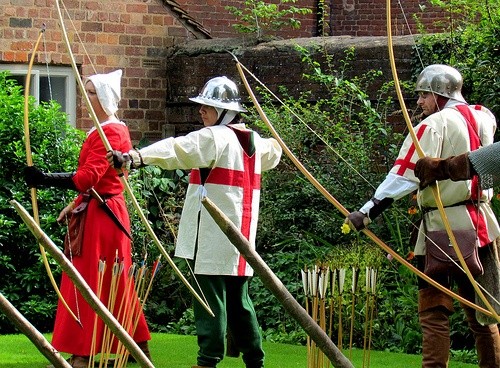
[413,153,472,191]
[343,211,371,236]
[23,165,77,192]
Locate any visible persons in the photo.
[23,69,153,368]
[106,75,283,368]
[344,64,500,368]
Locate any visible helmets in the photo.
[189,76,248,112]
[414,64,467,103]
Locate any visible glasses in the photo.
[417,91,432,98]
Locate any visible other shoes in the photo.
[123,354,151,363]
[66,355,93,368]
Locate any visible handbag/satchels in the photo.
[424,230,485,275]
